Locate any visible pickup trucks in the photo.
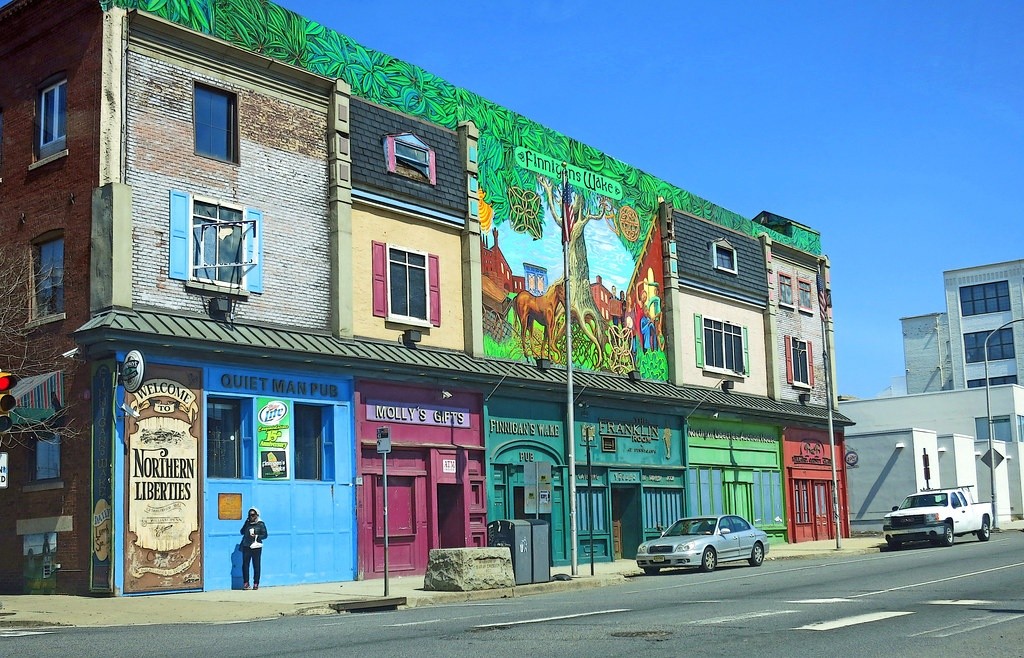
[881,489,992,547]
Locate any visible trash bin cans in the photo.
[486,517,550,586]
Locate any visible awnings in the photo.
[9,370,63,424]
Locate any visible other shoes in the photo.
[242,582,252,590]
[253,584,258,590]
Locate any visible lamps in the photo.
[721,380,734,393]
[398,329,422,350]
[626,370,641,382]
[798,393,810,402]
[207,295,236,320]
[533,356,550,371]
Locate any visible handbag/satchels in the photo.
[249,535,263,550]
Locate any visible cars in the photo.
[636,514,770,573]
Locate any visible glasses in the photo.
[249,514,255,517]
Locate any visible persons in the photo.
[240,506,268,590]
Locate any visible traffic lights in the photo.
[0,370,17,433]
